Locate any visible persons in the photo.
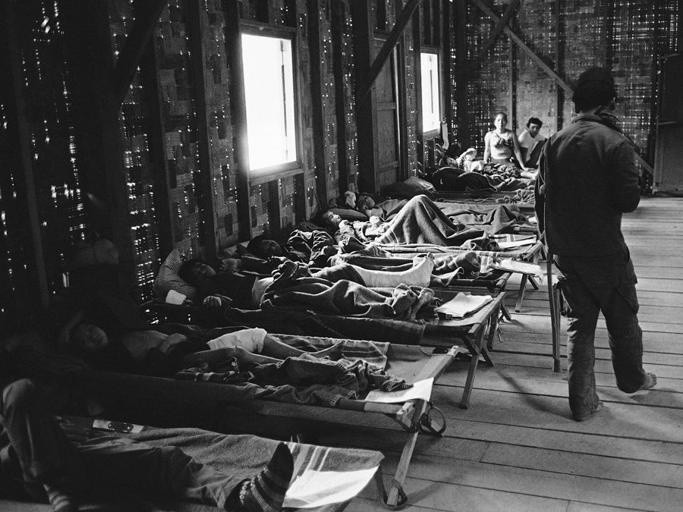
[1,193,552,512]
[534,67,657,422]
[420,111,549,198]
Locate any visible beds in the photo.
[0,413,385,511]
[219,245,521,351]
[293,231,542,312]
[1,322,462,511]
[379,189,537,213]
[473,159,540,180]
[143,273,506,409]
[328,200,551,258]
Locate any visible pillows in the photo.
[239,244,297,271]
[330,205,367,220]
[152,247,195,300]
[294,220,334,250]
[404,175,437,193]
